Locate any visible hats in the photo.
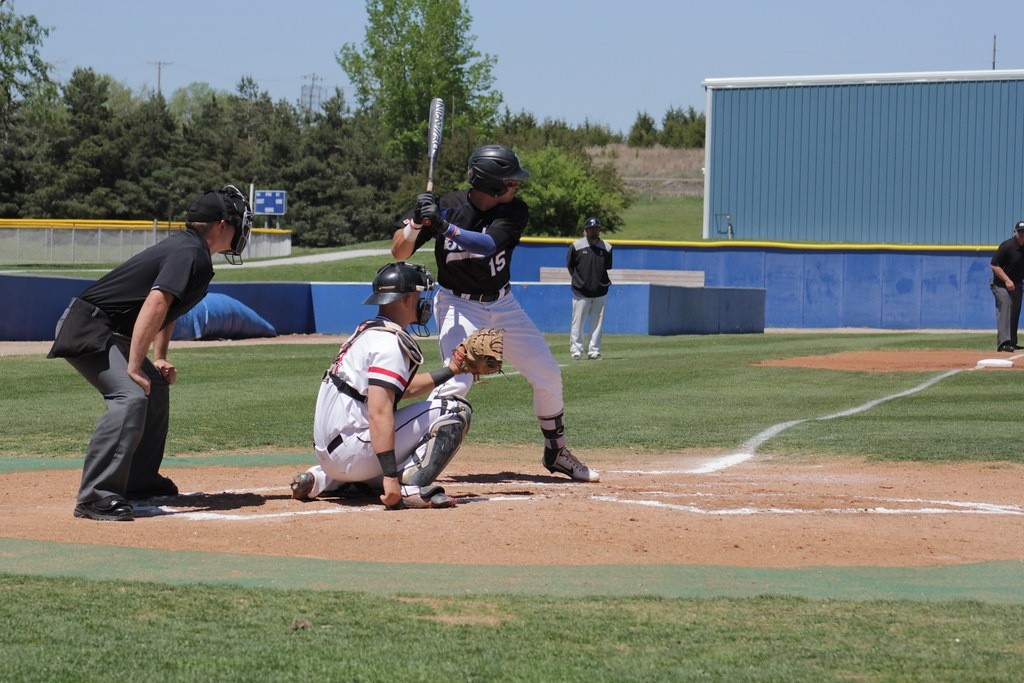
[1015,221,1024,232]
[186,189,245,227]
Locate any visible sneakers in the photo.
[289,472,315,499]
[542,447,601,482]
[998,342,1015,352]
[129,473,179,498]
[73,495,134,520]
[385,489,456,509]
[571,350,582,360]
[1011,343,1022,350]
[587,351,602,360]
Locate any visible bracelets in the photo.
[375,449,399,477]
[427,367,453,388]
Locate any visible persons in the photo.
[991,221,1024,352]
[390,143,600,508]
[565,216,614,360]
[290,261,504,507]
[55,183,252,523]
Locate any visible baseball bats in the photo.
[423,97,445,226]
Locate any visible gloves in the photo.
[420,204,449,235]
[410,191,441,230]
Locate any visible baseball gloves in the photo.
[451,326,514,384]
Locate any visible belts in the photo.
[451,283,512,303]
[313,435,343,455]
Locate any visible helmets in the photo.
[361,261,429,305]
[468,144,530,198]
[583,217,602,233]
